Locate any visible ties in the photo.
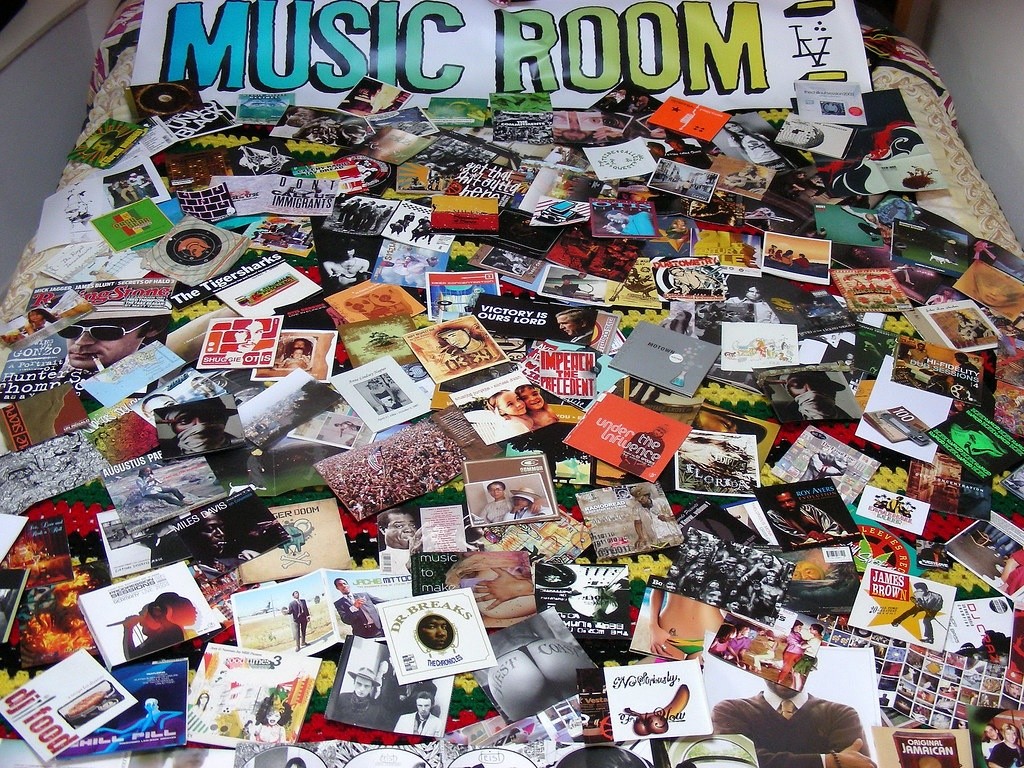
[299,606,302,614]
[781,700,795,719]
[347,595,368,624]
[418,721,424,735]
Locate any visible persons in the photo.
[41,87,1012,420]
[0,316,1023,768]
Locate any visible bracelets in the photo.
[830,750,841,767]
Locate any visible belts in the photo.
[363,624,375,629]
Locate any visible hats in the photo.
[780,373,845,399]
[348,667,381,688]
[510,488,542,504]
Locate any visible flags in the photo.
[368,446,382,471]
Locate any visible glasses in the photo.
[489,488,500,493]
[58,320,151,342]
[787,379,826,391]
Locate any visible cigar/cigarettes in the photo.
[92,355,105,372]
